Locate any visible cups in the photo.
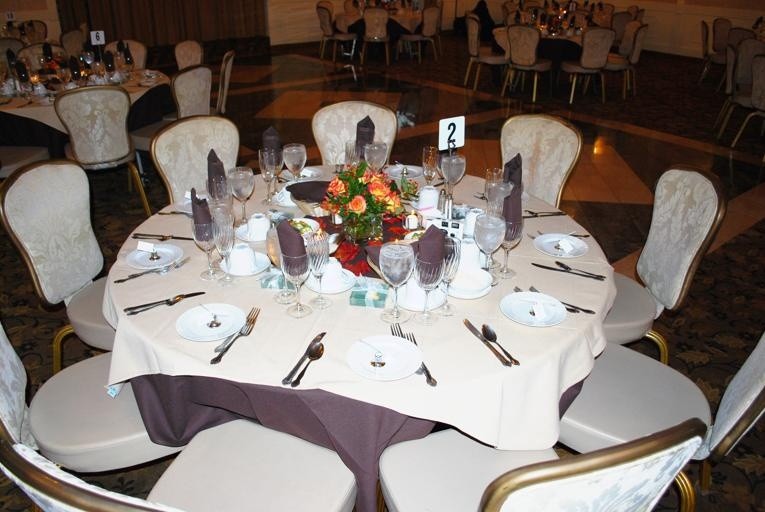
[515,9,584,36]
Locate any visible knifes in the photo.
[522,212,566,218]
[464,317,512,366]
[280,331,326,385]
[124,291,205,312]
[531,262,604,280]
[131,233,194,241]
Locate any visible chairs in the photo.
[1,159,118,376]
[316,1,765,99]
[311,101,399,172]
[151,114,240,204]
[1,100,226,197]
[601,164,729,363]
[554,335,765,512]
[500,114,582,208]
[2,418,358,510]
[1,328,191,474]
[0,19,233,99]
[378,419,707,511]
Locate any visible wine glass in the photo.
[191,139,525,324]
[6,40,136,101]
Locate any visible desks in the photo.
[103,163,619,452]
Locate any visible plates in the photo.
[174,196,192,213]
[126,244,183,270]
[533,233,590,257]
[346,335,423,381]
[500,290,568,328]
[175,303,247,342]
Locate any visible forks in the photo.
[157,210,193,217]
[402,332,437,386]
[113,256,191,283]
[526,230,589,240]
[515,288,580,314]
[392,323,423,376]
[529,286,595,313]
[210,308,260,365]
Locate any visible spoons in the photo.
[292,342,324,386]
[127,293,185,316]
[555,261,607,279]
[525,209,563,215]
[482,323,520,366]
